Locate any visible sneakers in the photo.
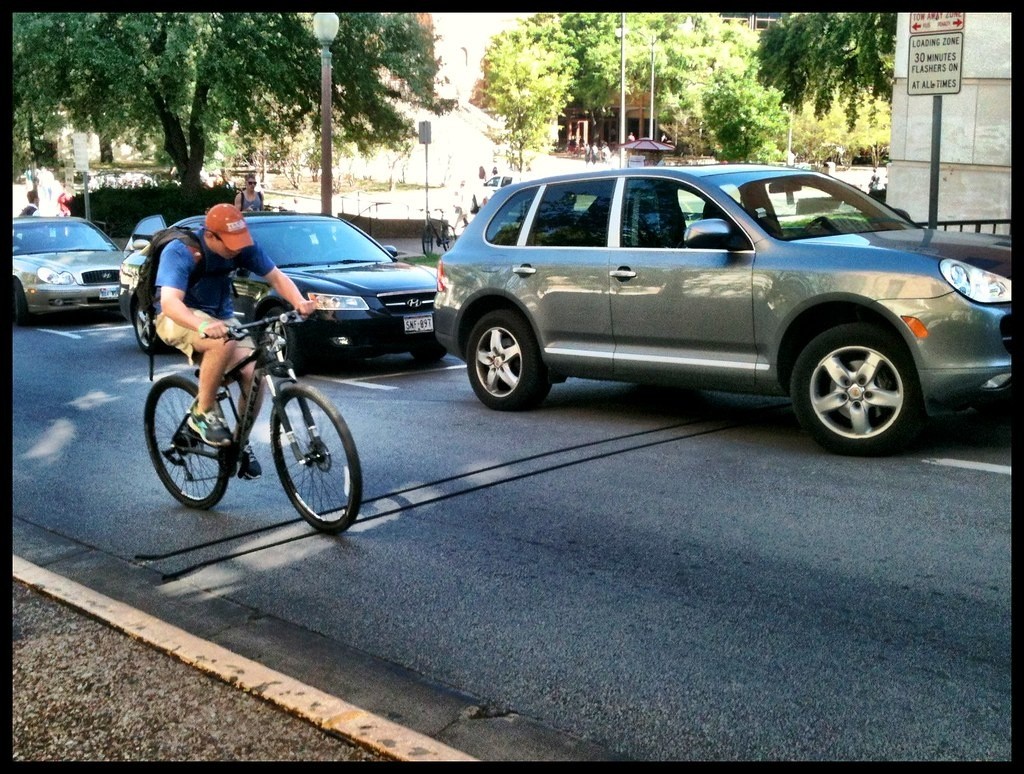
[187,401,232,445]
[230,431,263,479]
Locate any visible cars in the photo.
[117,212,448,380]
[12,213,167,327]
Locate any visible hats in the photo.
[206,203,254,252]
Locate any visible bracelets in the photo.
[197,321,207,333]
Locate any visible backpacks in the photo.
[19,206,36,216]
[134,225,206,381]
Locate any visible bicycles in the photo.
[143,308,364,536]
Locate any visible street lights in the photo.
[615,21,696,141]
[312,12,341,217]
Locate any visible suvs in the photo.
[432,163,1012,457]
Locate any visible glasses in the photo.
[248,182,257,185]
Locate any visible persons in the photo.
[627,130,635,141]
[234,172,264,211]
[660,132,667,142]
[152,202,315,478]
[448,164,501,231]
[869,167,881,190]
[573,135,613,164]
[20,184,78,217]
